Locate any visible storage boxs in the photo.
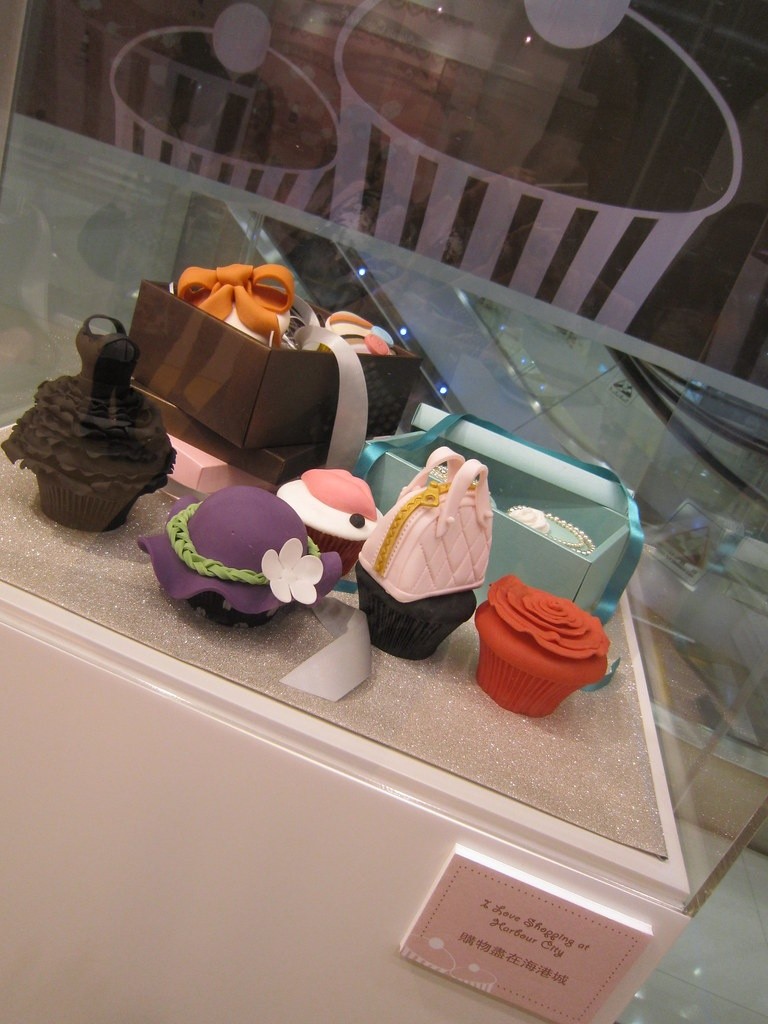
[167,433,297,495]
[353,405,635,611]
[129,379,329,486]
[128,279,425,450]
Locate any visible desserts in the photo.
[354,560,477,660]
[1,314,177,532]
[472,574,611,717]
[275,470,384,577]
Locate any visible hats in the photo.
[139,483,343,614]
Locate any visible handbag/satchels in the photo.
[358,447,499,604]
